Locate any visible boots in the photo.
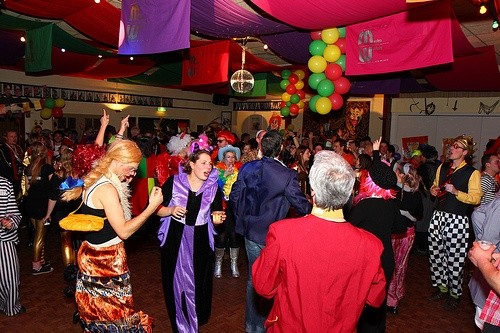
[230,247,240,278]
[214,247,225,278]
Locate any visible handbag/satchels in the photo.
[58,214,106,232]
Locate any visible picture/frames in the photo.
[0,79,174,108]
[220,110,232,131]
[233,101,285,111]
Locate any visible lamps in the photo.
[102,79,130,111]
[230,35,256,94]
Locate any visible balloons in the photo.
[308,27,351,115]
[281,70,306,117]
[40,98,66,118]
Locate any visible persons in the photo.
[63,140,163,333]
[0,130,25,185]
[229,130,312,333]
[468,189,500,333]
[427,134,485,312]
[205,126,435,315]
[0,176,26,317]
[154,131,227,333]
[252,149,387,333]
[22,108,175,281]
[473,153,500,211]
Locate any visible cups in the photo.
[213,211,223,224]
[479,241,492,251]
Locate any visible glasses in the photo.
[448,143,466,150]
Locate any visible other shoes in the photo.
[16,305,25,314]
[426,290,448,301]
[390,306,399,315]
[444,295,460,311]
[32,260,54,276]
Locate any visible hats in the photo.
[454,133,477,152]
[218,145,242,161]
